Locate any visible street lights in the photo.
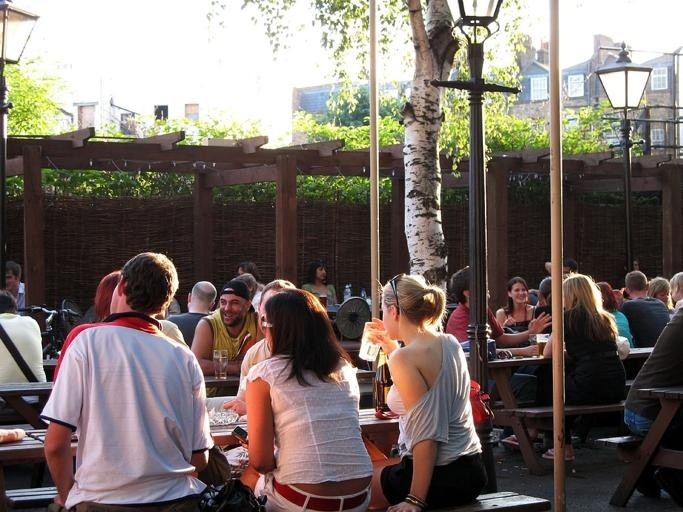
[426,0,522,496]
[593,40,655,274]
[0,0,43,290]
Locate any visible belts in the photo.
[273,476,374,512]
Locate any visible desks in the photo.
[1,301,400,508]
[442,304,681,507]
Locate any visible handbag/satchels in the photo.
[202,478,269,511]
[198,443,231,487]
[466,378,494,425]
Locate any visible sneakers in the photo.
[653,468,682,508]
[500,434,520,445]
[623,462,661,499]
[542,448,576,463]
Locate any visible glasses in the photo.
[389,271,405,317]
[260,316,273,331]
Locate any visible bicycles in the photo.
[15,304,84,361]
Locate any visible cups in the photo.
[357,319,380,363]
[210,350,230,381]
[486,338,497,360]
[318,295,328,307]
[535,334,549,356]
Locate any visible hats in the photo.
[219,280,252,303]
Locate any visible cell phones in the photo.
[232,426,248,445]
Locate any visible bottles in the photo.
[360,287,367,301]
[371,353,392,413]
[341,284,353,303]
